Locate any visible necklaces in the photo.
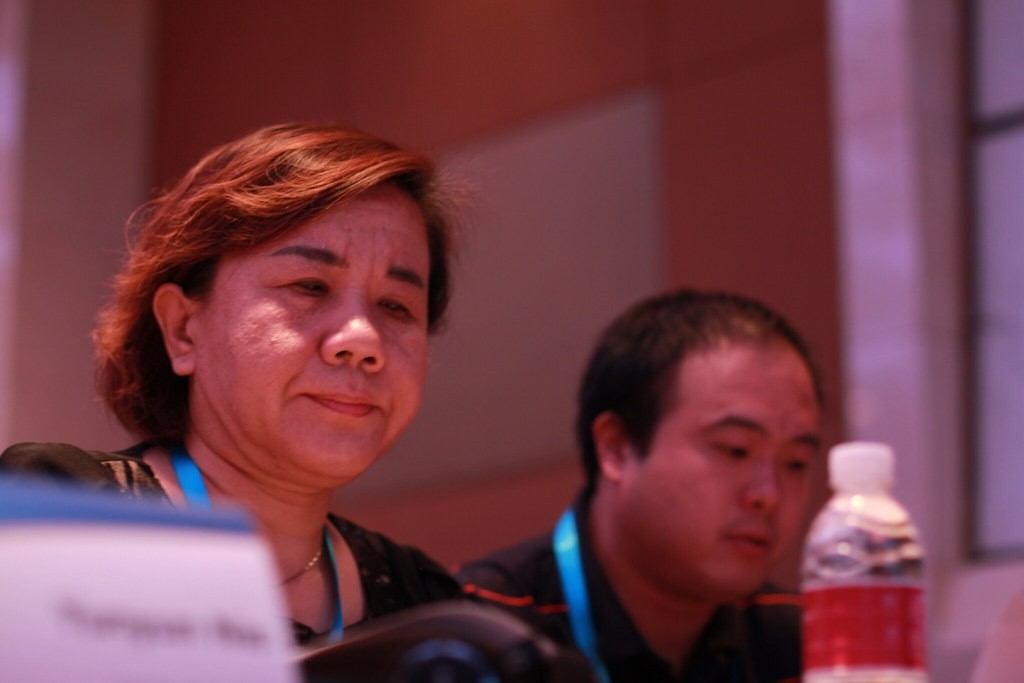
[281,551,321,587]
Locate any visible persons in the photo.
[0,122,464,653]
[452,287,825,683]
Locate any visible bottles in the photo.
[800,441,928,683]
